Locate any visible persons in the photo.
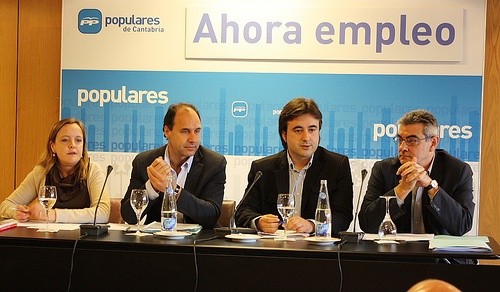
[120,102,227,229]
[358,111,476,237]
[235,97,354,235]
[0,118,111,224]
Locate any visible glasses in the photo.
[392,134,432,146]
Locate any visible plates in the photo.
[304,237,342,245]
[155,232,193,239]
[225,233,262,242]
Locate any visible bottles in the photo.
[160,174,178,232]
[314,180,332,240]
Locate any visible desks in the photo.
[0,219,500,292]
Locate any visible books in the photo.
[0,221,16,232]
[433,242,492,253]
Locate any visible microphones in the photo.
[214,171,262,238]
[80,165,113,236]
[338,169,368,243]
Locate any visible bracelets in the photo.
[174,185,181,196]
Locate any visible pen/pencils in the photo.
[274,220,284,223]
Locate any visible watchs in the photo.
[423,180,438,192]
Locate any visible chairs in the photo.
[108,198,126,223]
[217,201,237,226]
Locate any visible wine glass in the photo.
[378,195,398,240]
[38,186,57,232]
[276,194,295,237]
[127,189,150,235]
